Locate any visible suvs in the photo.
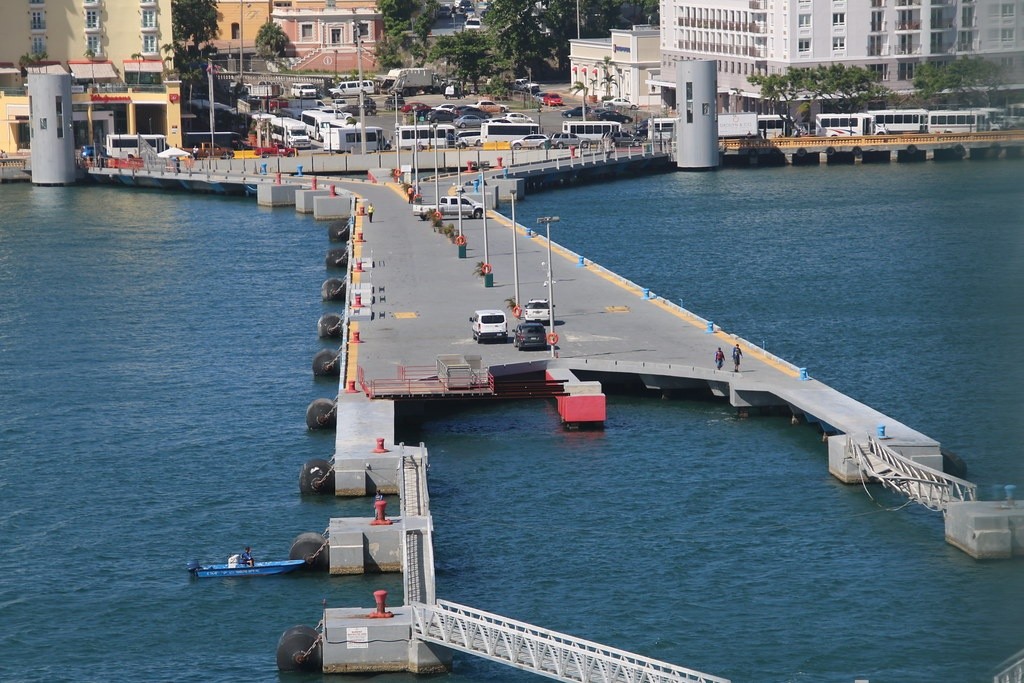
[512,323,547,351]
[525,298,556,323]
[81,145,107,160]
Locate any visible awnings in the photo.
[68,61,118,78]
[124,60,163,72]
[22,61,67,73]
[0,62,21,73]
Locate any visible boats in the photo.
[187,558,306,577]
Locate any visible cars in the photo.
[339,77,647,149]
[436,0,481,34]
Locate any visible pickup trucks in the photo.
[412,196,487,222]
[182,142,236,159]
[328,80,374,99]
[254,143,298,158]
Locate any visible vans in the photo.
[291,83,318,99]
[469,310,508,344]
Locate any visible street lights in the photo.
[478,168,491,276]
[537,216,563,357]
[509,188,524,306]
[390,90,464,245]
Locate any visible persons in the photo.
[407,186,414,204]
[0,149,8,158]
[384,140,391,150]
[794,129,800,135]
[368,203,374,223]
[731,344,742,372]
[176,157,181,173]
[193,146,199,160]
[242,547,254,567]
[715,347,726,370]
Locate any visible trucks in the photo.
[379,67,433,95]
[229,81,284,100]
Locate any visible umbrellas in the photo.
[157,147,190,159]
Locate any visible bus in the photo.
[562,119,621,144]
[253,100,392,153]
[644,101,1024,142]
[397,124,456,152]
[106,134,166,161]
[478,122,541,144]
